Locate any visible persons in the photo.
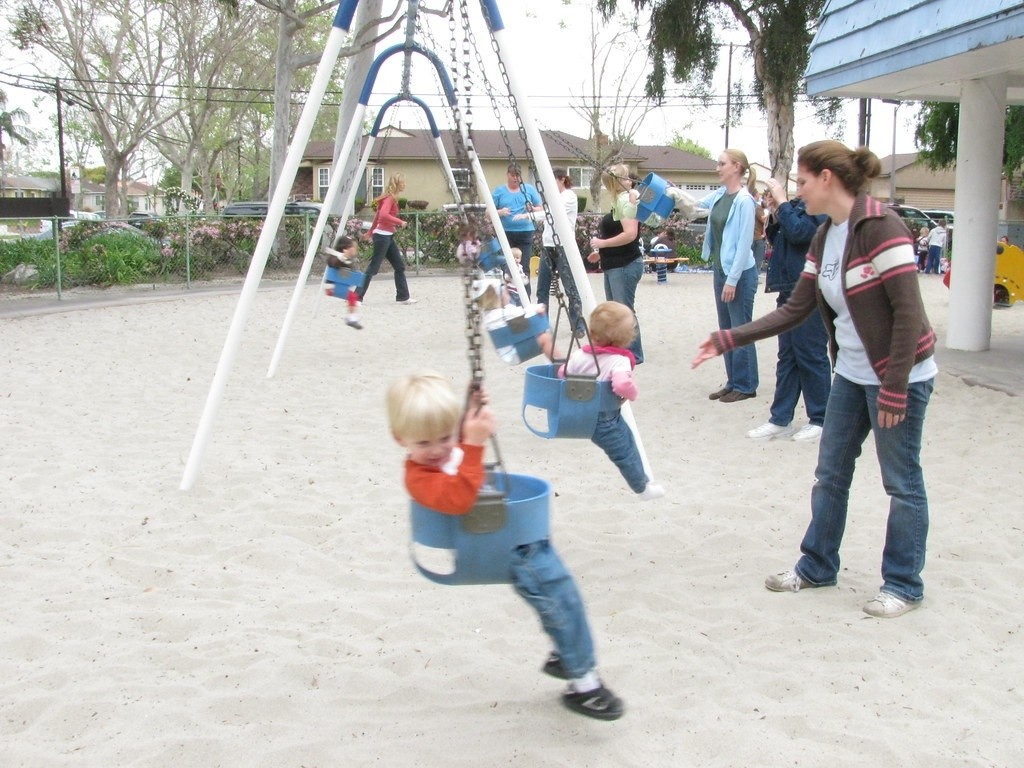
[472,279,563,366]
[323,236,364,330]
[354,173,418,307]
[457,222,481,266]
[750,187,768,271]
[383,371,624,722]
[892,203,913,234]
[745,179,831,442]
[587,170,644,366]
[602,163,709,223]
[1000,236,1009,244]
[915,227,929,272]
[558,300,666,501]
[692,139,939,617]
[492,163,543,308]
[667,149,759,403]
[646,226,678,273]
[512,165,585,339]
[762,190,779,223]
[502,248,529,306]
[920,219,947,274]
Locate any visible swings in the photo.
[411,0,680,588]
[323,92,405,299]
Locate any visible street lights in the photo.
[881,98,903,205]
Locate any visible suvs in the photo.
[220,200,373,249]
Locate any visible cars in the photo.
[127,212,162,229]
[24,210,108,245]
[907,210,955,231]
[883,203,939,236]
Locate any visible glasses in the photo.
[713,161,725,168]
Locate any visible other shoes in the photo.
[638,483,666,500]
[345,312,363,330]
[688,207,709,221]
[708,389,730,400]
[396,297,418,305]
[719,391,756,402]
[575,326,585,338]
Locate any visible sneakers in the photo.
[863,591,923,619]
[544,652,567,680]
[563,685,623,720]
[790,423,823,440]
[765,567,814,592]
[746,422,789,439]
